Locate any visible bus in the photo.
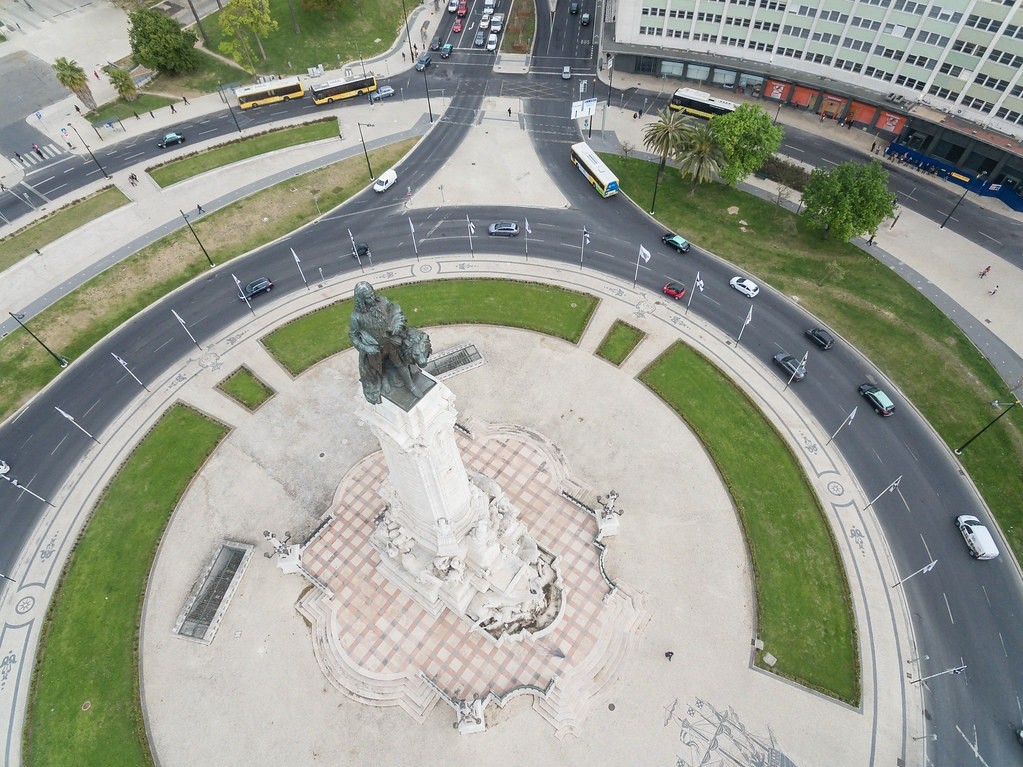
[670,86,741,122]
[569,141,620,199]
[582,14,589,26]
[235,75,304,111]
[308,71,378,106]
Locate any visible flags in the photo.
[889,477,902,492]
[171,309,186,324]
[799,352,808,370]
[112,353,127,365]
[291,248,301,263]
[640,245,651,263]
[525,218,531,234]
[745,305,753,325]
[849,407,858,425]
[348,229,355,244]
[948,666,967,674]
[467,215,476,234]
[0,407,74,488]
[697,272,704,291]
[232,274,240,284]
[924,560,938,574]
[409,217,415,233]
[584,227,590,246]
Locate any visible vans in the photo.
[372,168,398,194]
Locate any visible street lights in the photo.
[67,122,109,179]
[179,207,214,266]
[956,399,1017,453]
[646,156,663,214]
[216,79,242,133]
[940,170,988,228]
[8,311,67,368]
[422,64,439,122]
[356,122,375,180]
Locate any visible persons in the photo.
[633,108,643,119]
[0,70,204,214]
[819,112,1000,296]
[508,107,512,117]
[348,281,424,400]
[402,44,417,62]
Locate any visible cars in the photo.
[774,350,808,382]
[365,85,395,101]
[661,232,691,254]
[729,276,760,298]
[858,382,897,417]
[805,326,835,350]
[351,242,370,257]
[0,459,12,480]
[662,281,686,300]
[156,130,187,148]
[570,2,579,14]
[1016,726,1023,747]
[956,514,1000,561]
[488,222,521,237]
[235,276,274,302]
[415,0,505,72]
[562,66,572,79]
